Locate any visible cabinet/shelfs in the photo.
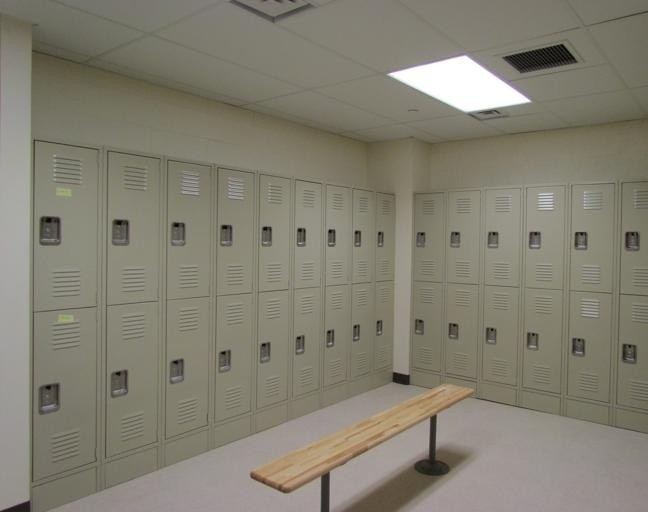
[31,138,398,511]
[410,178,648,434]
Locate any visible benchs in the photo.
[239,377,479,511]
